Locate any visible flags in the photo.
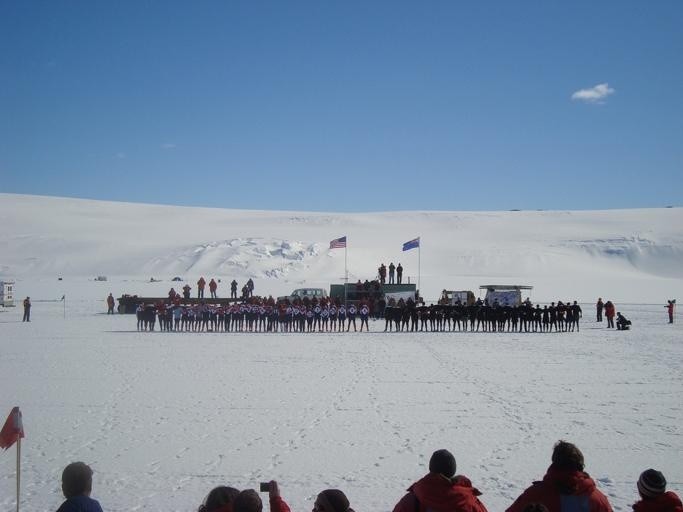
[5,411,23,450]
[328,235,344,249]
[0,406,24,452]
[401,238,418,251]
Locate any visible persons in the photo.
[311,488,356,512]
[183,284,191,298]
[377,263,387,284]
[197,485,240,511]
[603,300,616,328]
[105,292,116,314]
[119,293,138,313]
[388,295,415,307]
[209,278,217,299]
[663,298,677,324]
[233,479,290,511]
[630,468,683,511]
[55,461,105,511]
[197,277,206,299]
[442,289,448,304]
[504,438,615,511]
[21,296,33,323]
[392,448,488,512]
[385,297,582,333]
[596,297,604,322]
[388,262,395,285]
[396,262,403,285]
[135,295,370,333]
[231,280,238,299]
[415,289,419,302]
[615,312,632,330]
[355,279,370,300]
[368,296,386,320]
[168,287,176,305]
[242,278,254,299]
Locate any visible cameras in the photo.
[260,483,270,492]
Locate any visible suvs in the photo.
[276,287,327,305]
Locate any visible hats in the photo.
[429,450,455,474]
[636,468,666,497]
[314,489,349,512]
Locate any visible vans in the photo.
[437,291,475,306]
